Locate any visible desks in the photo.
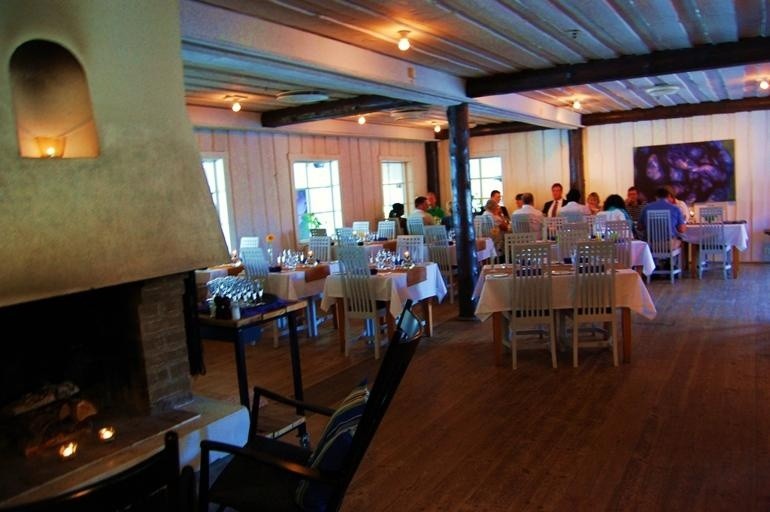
[195,290,308,437]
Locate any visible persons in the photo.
[390,183,689,278]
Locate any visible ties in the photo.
[552,200,558,217]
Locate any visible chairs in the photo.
[193,201,746,371]
[200,298,426,510]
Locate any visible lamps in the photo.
[757,75,769,90]
[572,94,581,110]
[433,121,443,133]
[645,85,678,97]
[398,29,410,51]
[357,113,368,125]
[226,94,245,112]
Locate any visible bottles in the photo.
[376,248,400,269]
[277,248,304,270]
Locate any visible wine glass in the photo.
[207,277,265,308]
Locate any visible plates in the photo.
[493,273,508,277]
[552,270,568,275]
